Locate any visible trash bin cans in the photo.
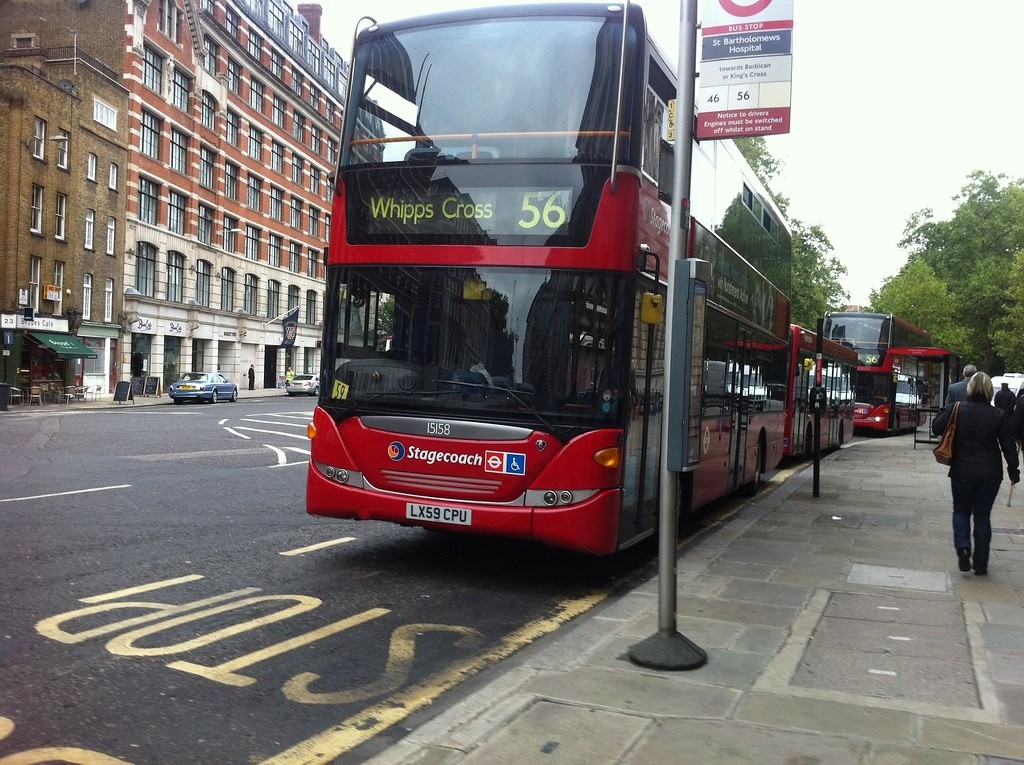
[0,383,11,411]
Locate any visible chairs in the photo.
[29,387,42,406]
[59,386,76,405]
[9,387,25,406]
[49,383,64,403]
[75,387,89,403]
[87,385,102,402]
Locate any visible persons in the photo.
[945,365,977,405]
[932,372,1020,576]
[416,316,482,372]
[248,364,255,390]
[285,367,294,393]
[994,383,1024,459]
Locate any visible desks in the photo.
[22,388,47,405]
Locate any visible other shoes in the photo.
[959,548,971,572]
[249,388,255,390]
[975,565,987,576]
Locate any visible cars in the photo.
[286,374,320,397]
[168,372,239,404]
[781,323,858,469]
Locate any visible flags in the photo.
[279,308,299,348]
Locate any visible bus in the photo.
[305,2,791,557]
[822,310,932,433]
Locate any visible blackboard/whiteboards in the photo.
[113,381,131,402]
[143,375,160,395]
[130,376,144,395]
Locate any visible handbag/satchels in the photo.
[933,401,960,465]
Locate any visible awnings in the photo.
[29,333,98,359]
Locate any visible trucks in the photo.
[989,372,1024,407]
